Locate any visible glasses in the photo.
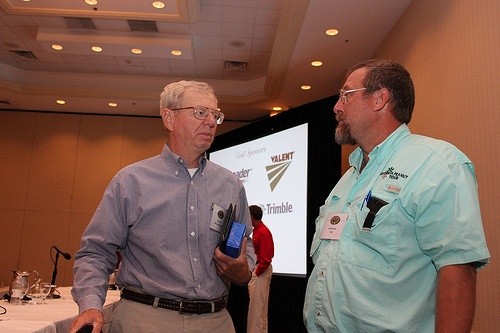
[339,83,383,106]
[171,106,225,125]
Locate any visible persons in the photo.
[68,80,275,333]
[303,56,492,333]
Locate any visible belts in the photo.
[120,289,228,315]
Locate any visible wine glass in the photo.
[37,283,51,305]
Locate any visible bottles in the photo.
[21,272,29,291]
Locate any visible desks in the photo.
[0,286,121,333]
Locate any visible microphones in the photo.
[53,246,71,260]
